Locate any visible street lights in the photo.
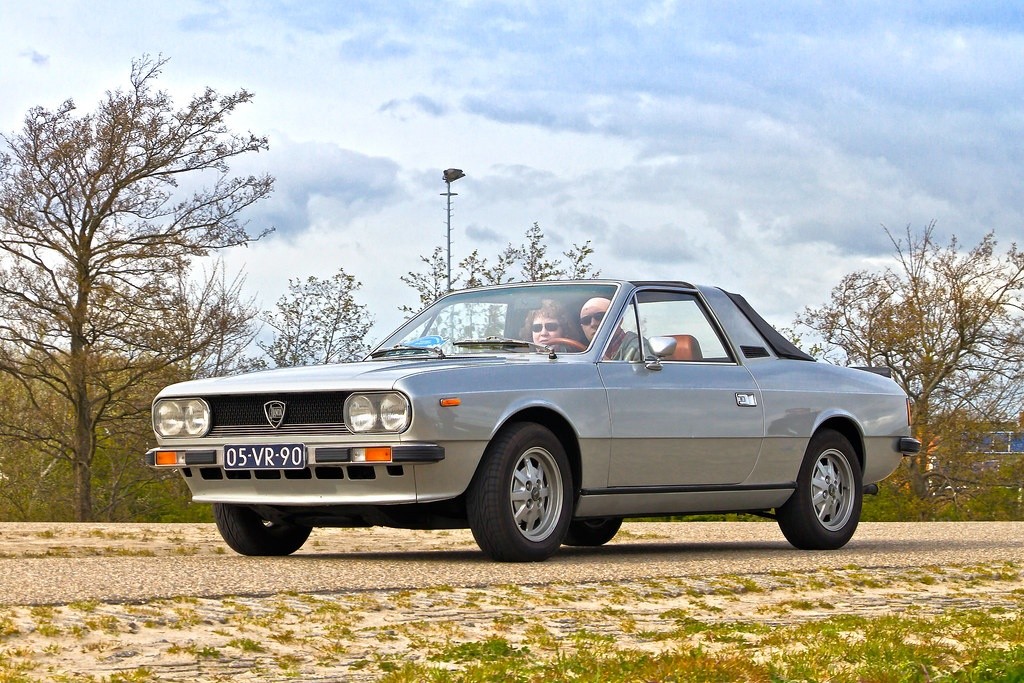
[440,168,466,292]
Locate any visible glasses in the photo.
[530,322,559,332]
[578,311,606,325]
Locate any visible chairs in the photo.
[661,335,703,361]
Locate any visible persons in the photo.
[579,296,660,361]
[519,299,585,353]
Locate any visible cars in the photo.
[143,279,920,564]
